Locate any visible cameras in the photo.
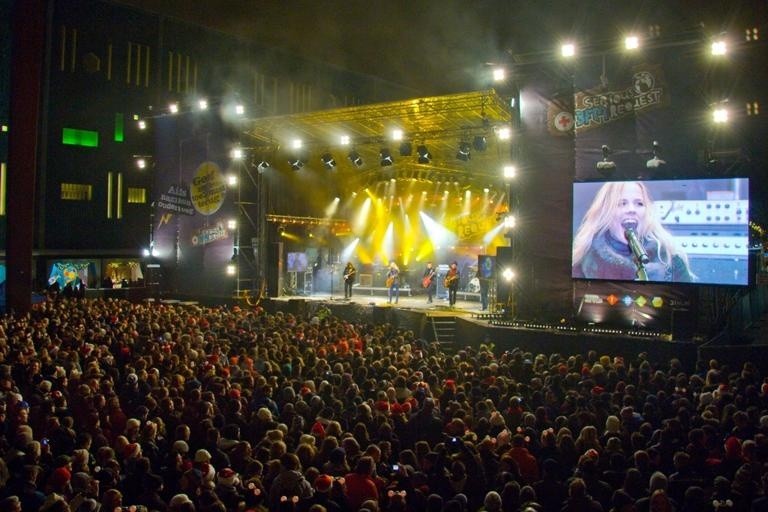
[43,439,50,446]
[451,437,457,443]
[518,398,523,402]
[324,371,330,375]
[391,463,399,471]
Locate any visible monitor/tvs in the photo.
[288,252,307,272]
[478,255,496,279]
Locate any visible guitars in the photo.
[422,272,435,287]
[344,271,355,280]
[385,272,398,288]
[444,275,458,287]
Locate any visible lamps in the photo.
[255,117,491,174]
[645,141,671,177]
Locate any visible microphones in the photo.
[328,268,334,275]
[624,229,650,264]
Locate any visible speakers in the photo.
[144,264,161,287]
[269,242,284,298]
[496,246,512,303]
[436,264,451,300]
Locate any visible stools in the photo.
[493,302,507,314]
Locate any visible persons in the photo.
[446,260,461,305]
[385,262,401,304]
[1,280,768,511]
[573,181,699,283]
[474,270,490,312]
[645,147,673,173]
[343,262,356,298]
[422,261,437,305]
[293,253,302,271]
